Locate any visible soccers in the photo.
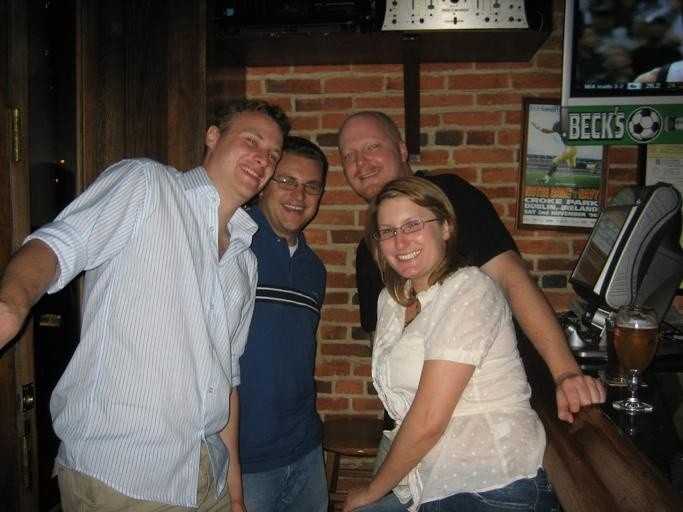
[628,108,662,141]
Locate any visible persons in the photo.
[337,110,606,479]
[342,175,553,512]
[238,135,329,512]
[0,99,291,512]
[576,0,683,83]
[531,121,597,183]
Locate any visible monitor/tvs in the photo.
[571,201,636,292]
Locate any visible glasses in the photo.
[372,218,439,240]
[270,177,325,195]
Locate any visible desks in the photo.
[513,312,681,512]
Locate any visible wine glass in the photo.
[613,305,660,413]
